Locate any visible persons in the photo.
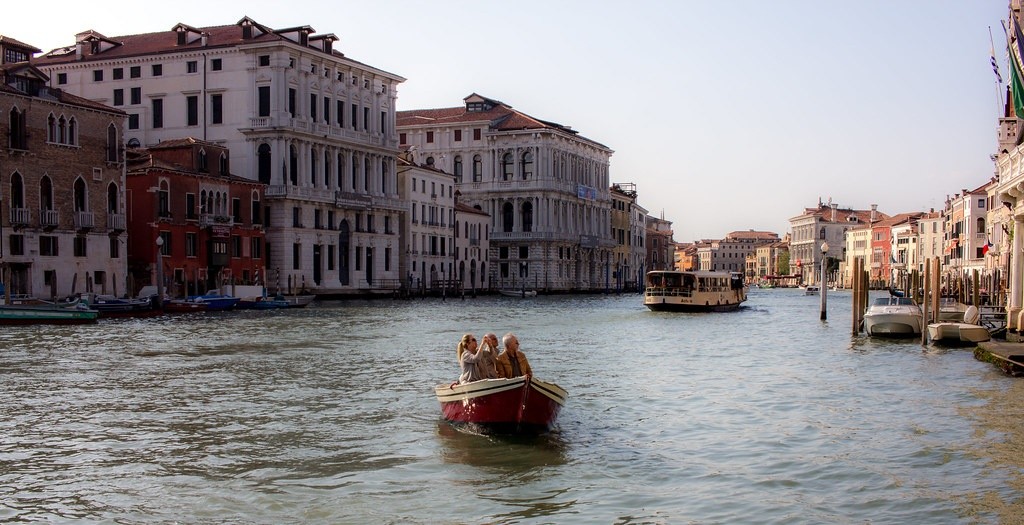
[484,332,533,379]
[456,334,494,383]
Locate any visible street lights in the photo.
[522,261,526,299]
[820,242,830,321]
[155,236,164,297]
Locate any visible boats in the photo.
[921,297,991,344]
[434,372,569,435]
[805,285,821,296]
[863,295,923,340]
[756,275,799,289]
[643,268,748,313]
[499,289,536,298]
[0,284,316,325]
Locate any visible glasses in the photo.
[468,338,476,343]
